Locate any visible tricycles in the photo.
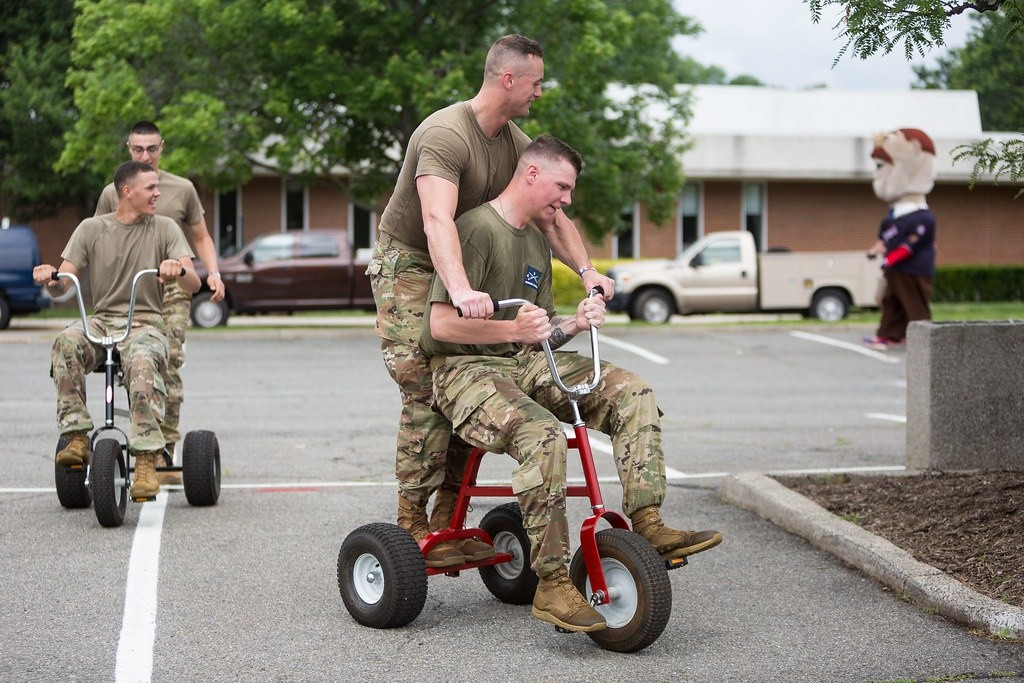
[51,268,223,527]
[335,285,671,653]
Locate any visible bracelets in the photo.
[209,270,221,279]
[579,265,596,276]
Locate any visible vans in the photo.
[0,226,53,330]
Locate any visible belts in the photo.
[375,230,429,255]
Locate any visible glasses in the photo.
[128,140,162,151]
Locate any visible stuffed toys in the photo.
[860,127,939,351]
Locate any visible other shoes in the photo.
[863,335,906,351]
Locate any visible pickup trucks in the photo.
[604,230,886,325]
[190,228,376,329]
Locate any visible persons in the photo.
[417,134,723,632]
[364,33,615,568]
[92,120,226,492]
[33,160,202,498]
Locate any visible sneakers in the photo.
[55,431,90,467]
[532,563,608,633]
[130,452,161,497]
[155,447,182,485]
[396,489,494,567]
[631,501,722,561]
